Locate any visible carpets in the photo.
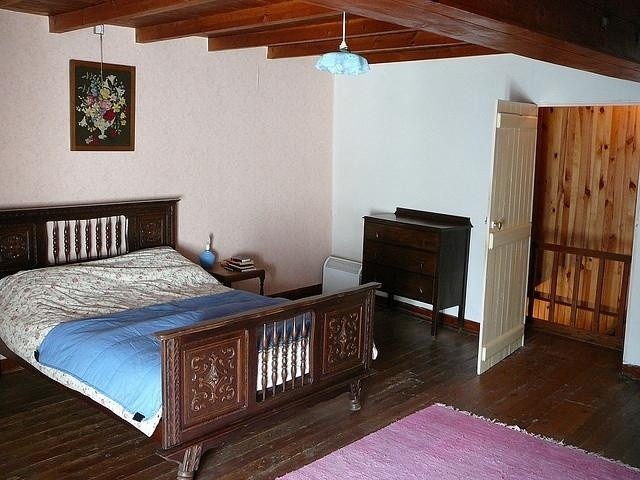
[275,402,639,479]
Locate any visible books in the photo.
[225,254,257,272]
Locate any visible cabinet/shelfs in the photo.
[361,206,475,342]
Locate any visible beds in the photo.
[0,196,383,479]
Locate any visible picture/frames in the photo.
[69,59,136,151]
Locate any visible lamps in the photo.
[314,9,370,76]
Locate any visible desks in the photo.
[208,262,266,296]
